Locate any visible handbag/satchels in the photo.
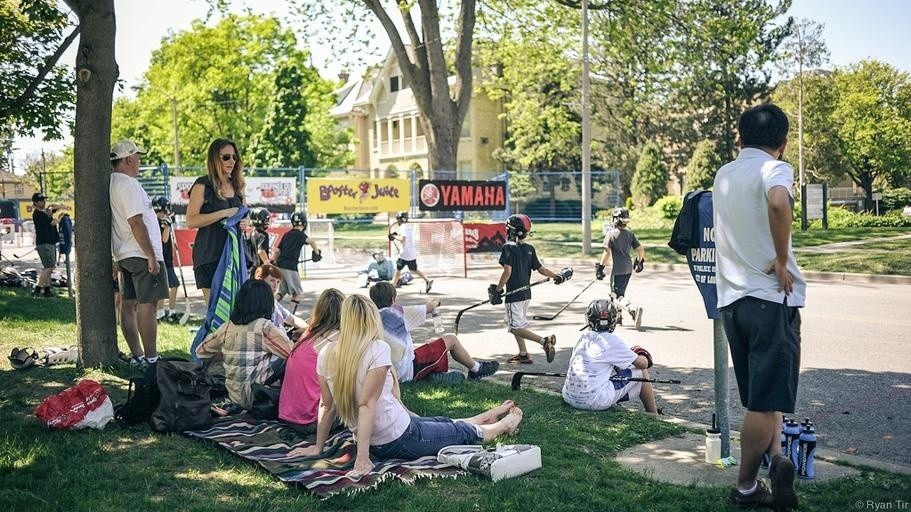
[250,383,281,421]
[437,442,542,483]
[114,357,213,431]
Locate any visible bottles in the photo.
[760,413,818,481]
[432,307,446,334]
[702,413,722,466]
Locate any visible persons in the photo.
[596,206,644,330]
[277,288,420,434]
[562,300,658,412]
[286,293,524,472]
[368,280,501,385]
[360,249,413,289]
[188,139,322,414]
[105,139,180,378]
[31,194,65,300]
[488,213,557,364]
[705,104,807,511]
[389,212,433,294]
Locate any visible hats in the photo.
[32,193,47,201]
[110,140,146,160]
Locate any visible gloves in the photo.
[631,344,653,368]
[161,211,176,228]
[633,257,644,273]
[388,232,397,240]
[595,262,605,280]
[312,249,322,262]
[553,273,565,285]
[488,284,504,305]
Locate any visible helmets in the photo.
[504,214,532,241]
[152,195,171,213]
[371,248,384,260]
[291,211,307,230]
[249,207,271,230]
[586,300,618,332]
[396,211,408,225]
[611,208,630,228]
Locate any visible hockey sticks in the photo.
[0,254,14,266]
[533,277,598,320]
[166,209,191,325]
[388,222,400,253]
[13,249,35,259]
[511,372,682,391]
[454,277,554,336]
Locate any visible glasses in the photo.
[219,153,237,161]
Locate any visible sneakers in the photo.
[128,355,162,373]
[634,306,643,329]
[428,371,466,384]
[466,360,499,381]
[426,280,434,293]
[768,453,799,510]
[156,313,178,325]
[731,480,774,505]
[30,291,56,298]
[509,354,533,363]
[542,334,556,362]
